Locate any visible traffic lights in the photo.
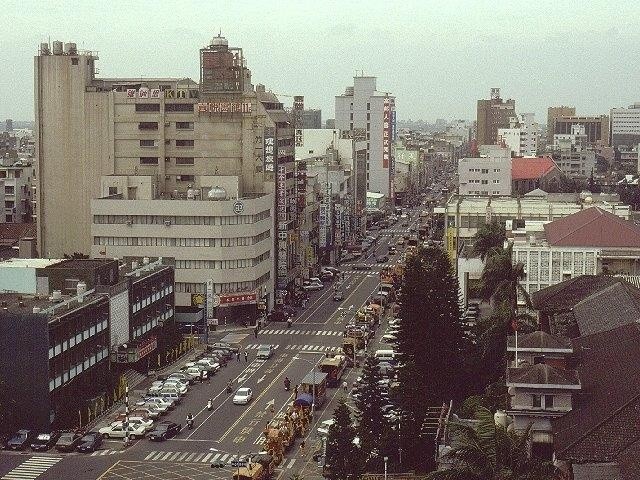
[210,462,225,469]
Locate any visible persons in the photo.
[331,350,335,358]
[236,351,241,362]
[342,380,348,391]
[284,377,291,390]
[258,319,261,328]
[124,435,129,446]
[227,379,234,391]
[186,412,194,424]
[244,351,249,362]
[326,348,329,358]
[254,327,258,338]
[287,317,293,326]
[292,385,299,400]
[300,441,305,454]
[270,405,275,418]
[208,396,213,406]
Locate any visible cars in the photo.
[301,277,324,292]
[7,428,104,452]
[377,256,389,262]
[315,267,340,282]
[256,345,274,359]
[333,291,343,301]
[98,341,240,440]
[352,263,371,270]
[233,388,253,405]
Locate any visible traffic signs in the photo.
[231,461,247,467]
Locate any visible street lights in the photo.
[293,356,315,433]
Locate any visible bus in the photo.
[374,350,414,362]
